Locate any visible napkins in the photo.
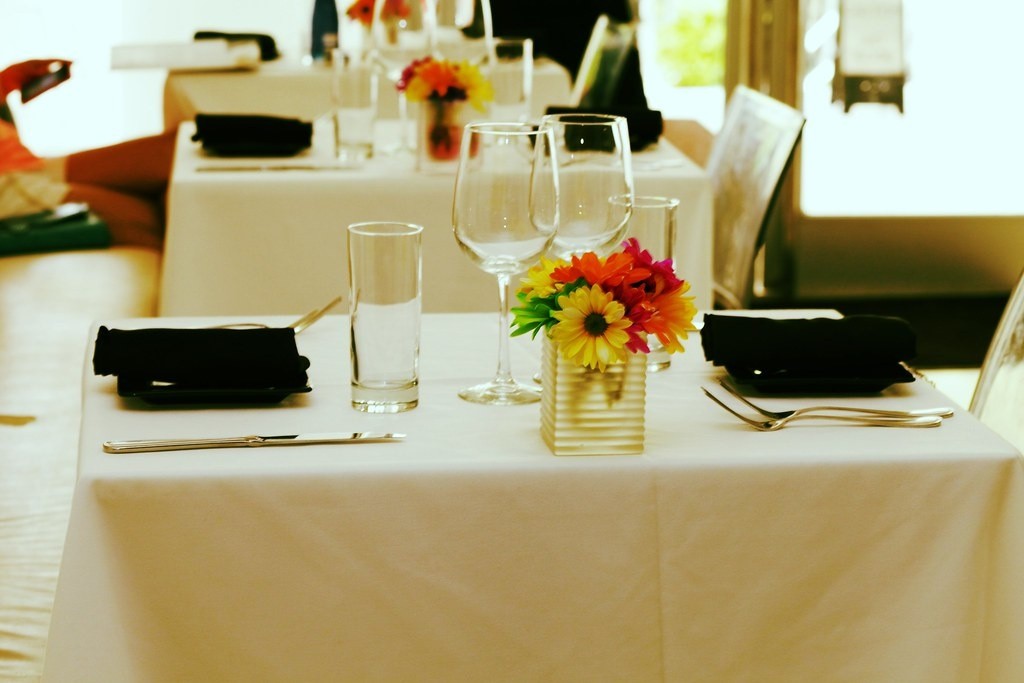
[191,113,312,146]
[545,107,663,137]
[93,326,310,385]
[701,314,917,366]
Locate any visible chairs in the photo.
[565,13,632,112]
[700,84,806,310]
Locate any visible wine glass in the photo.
[453,123,561,406]
[336,0,493,158]
[530,114,635,383]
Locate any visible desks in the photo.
[160,115,713,317]
[46,310,1024,683]
[160,56,571,131]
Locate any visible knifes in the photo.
[103,432,407,453]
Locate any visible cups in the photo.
[332,49,381,160]
[609,196,679,371]
[347,221,423,414]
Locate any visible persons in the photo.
[461,0,653,130]
[0,52,190,248]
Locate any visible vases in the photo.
[540,327,649,456]
[384,20,400,44]
[423,100,463,160]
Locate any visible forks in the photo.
[701,386,943,431]
[717,378,954,418]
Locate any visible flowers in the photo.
[395,55,496,115]
[379,0,411,22]
[509,236,699,374]
[346,0,376,22]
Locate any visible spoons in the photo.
[217,296,341,335]
